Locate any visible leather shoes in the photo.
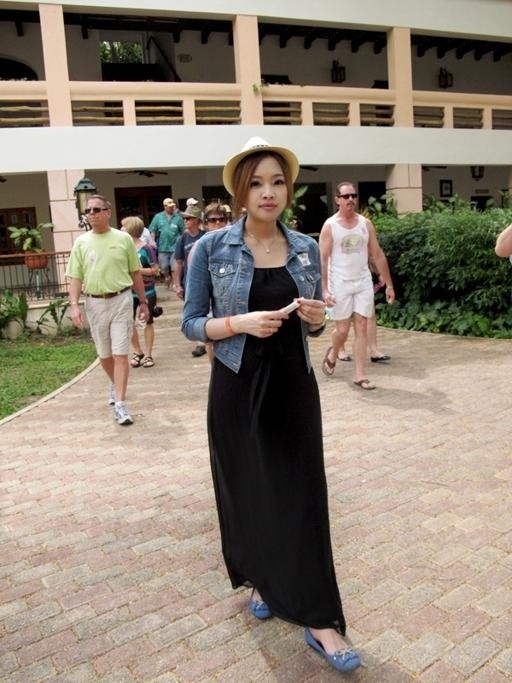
[249,586,273,620]
[337,352,352,361]
[371,354,393,363]
[305,626,360,672]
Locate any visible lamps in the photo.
[438,66,453,89]
[471,166,484,181]
[330,57,347,83]
[74,174,98,232]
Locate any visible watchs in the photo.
[139,299,148,304]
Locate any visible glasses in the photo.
[85,208,109,214]
[208,216,225,222]
[338,193,357,199]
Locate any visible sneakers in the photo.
[192,345,207,355]
[131,353,154,366]
[114,403,133,424]
[165,276,177,292]
[110,384,115,405]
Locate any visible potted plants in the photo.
[0,286,28,340]
[9,222,55,270]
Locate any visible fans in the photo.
[117,170,169,178]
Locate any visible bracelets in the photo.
[224,316,235,335]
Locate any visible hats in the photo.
[163,198,232,220]
[223,136,299,199]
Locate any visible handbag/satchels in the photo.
[132,275,154,292]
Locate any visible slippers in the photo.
[323,348,335,376]
[354,379,376,389]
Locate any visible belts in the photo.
[85,287,129,298]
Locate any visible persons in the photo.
[180,135,365,676]
[204,202,227,229]
[65,194,149,425]
[494,222,512,263]
[319,182,395,391]
[174,206,208,356]
[223,204,232,224]
[148,197,184,287]
[186,197,201,205]
[338,306,390,362]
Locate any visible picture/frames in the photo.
[439,178,453,197]
